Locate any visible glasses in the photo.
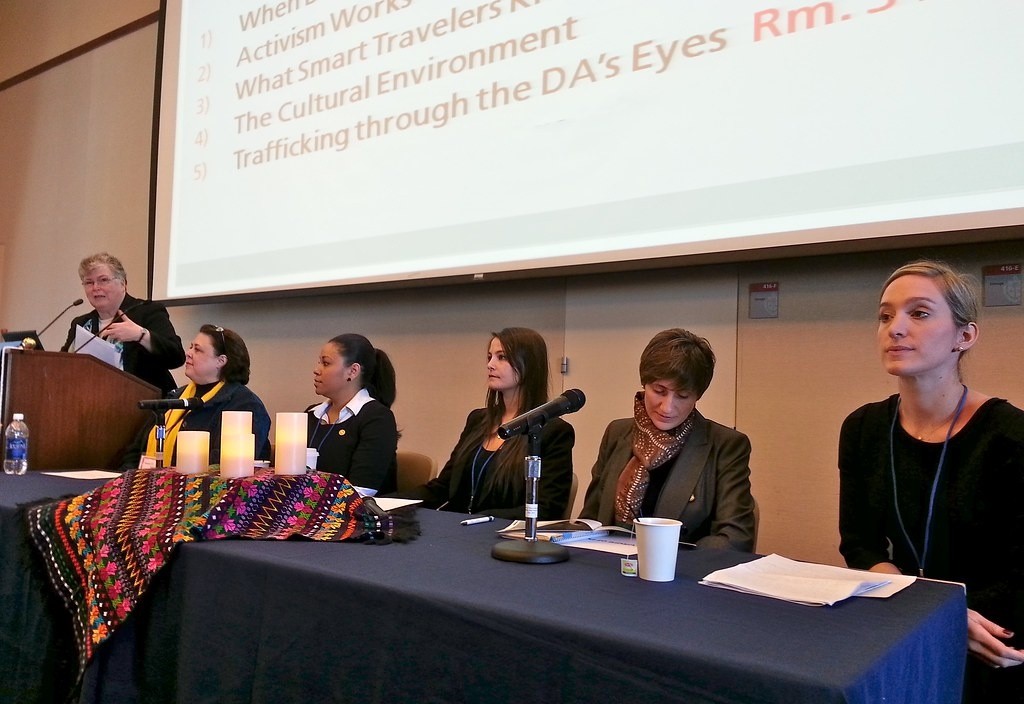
[210,325,225,349]
[82,277,120,288]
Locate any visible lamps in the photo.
[176,431,210,475]
[219,411,256,479]
[275,412,308,475]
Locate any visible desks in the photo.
[0,468,971,704]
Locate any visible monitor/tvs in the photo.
[2,330,45,351]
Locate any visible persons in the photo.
[58,252,186,400]
[399,327,576,523]
[837,261,1024,704]
[576,327,757,554]
[305,333,401,495]
[118,324,271,473]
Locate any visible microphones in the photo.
[75,298,153,352]
[497,389,586,439]
[32,299,84,342]
[137,397,204,411]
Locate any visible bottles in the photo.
[3,414,29,475]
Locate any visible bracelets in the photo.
[138,328,146,344]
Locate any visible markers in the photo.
[461,516,495,526]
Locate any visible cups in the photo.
[307,448,318,470]
[633,517,683,582]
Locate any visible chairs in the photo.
[395,450,438,497]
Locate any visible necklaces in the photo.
[309,403,347,451]
[890,385,968,579]
